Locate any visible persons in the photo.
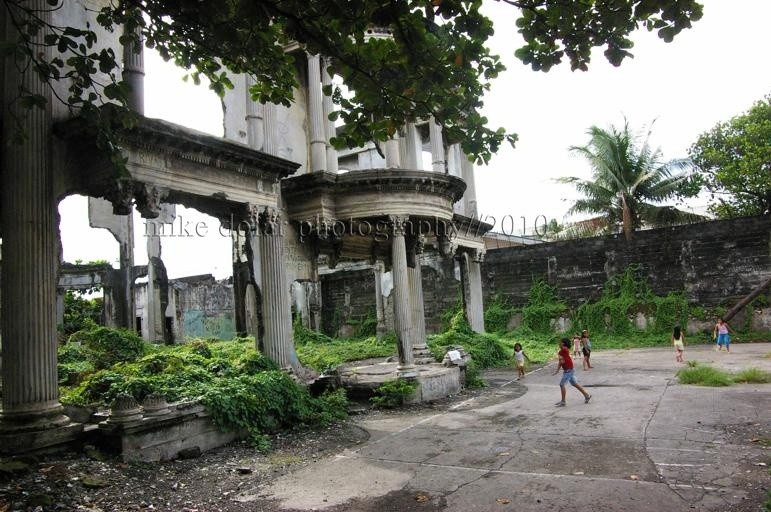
[581,329,595,371]
[509,342,530,382]
[713,317,736,353]
[550,338,592,408]
[572,331,583,360]
[670,325,686,363]
